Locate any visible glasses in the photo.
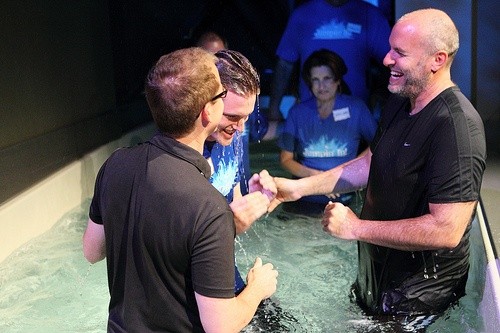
[196,86,228,121]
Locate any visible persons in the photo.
[268,0,392,120]
[200,32,278,204]
[82,46,279,333]
[267,9,487,333]
[203,49,278,298]
[273,49,378,218]
